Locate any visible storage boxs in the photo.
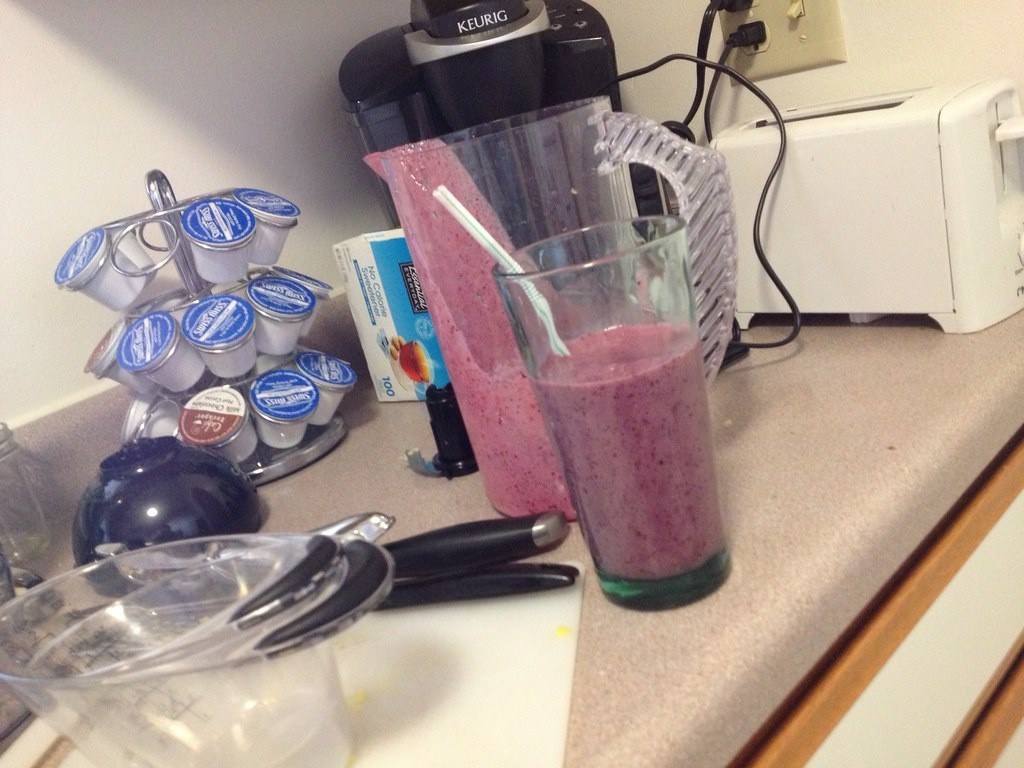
[334,229,451,402]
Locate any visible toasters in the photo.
[708,78,1023,332]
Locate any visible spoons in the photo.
[427,185,570,356]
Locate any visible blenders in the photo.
[336,2,625,223]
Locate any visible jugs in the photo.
[361,94,733,521]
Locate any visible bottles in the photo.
[0,423,50,565]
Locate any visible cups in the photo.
[53,187,355,464]
[490,216,731,612]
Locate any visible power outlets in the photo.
[716,0,849,85]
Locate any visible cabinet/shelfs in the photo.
[0,311,1024,768]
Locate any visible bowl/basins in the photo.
[72,435,260,597]
[0,533,395,767]
